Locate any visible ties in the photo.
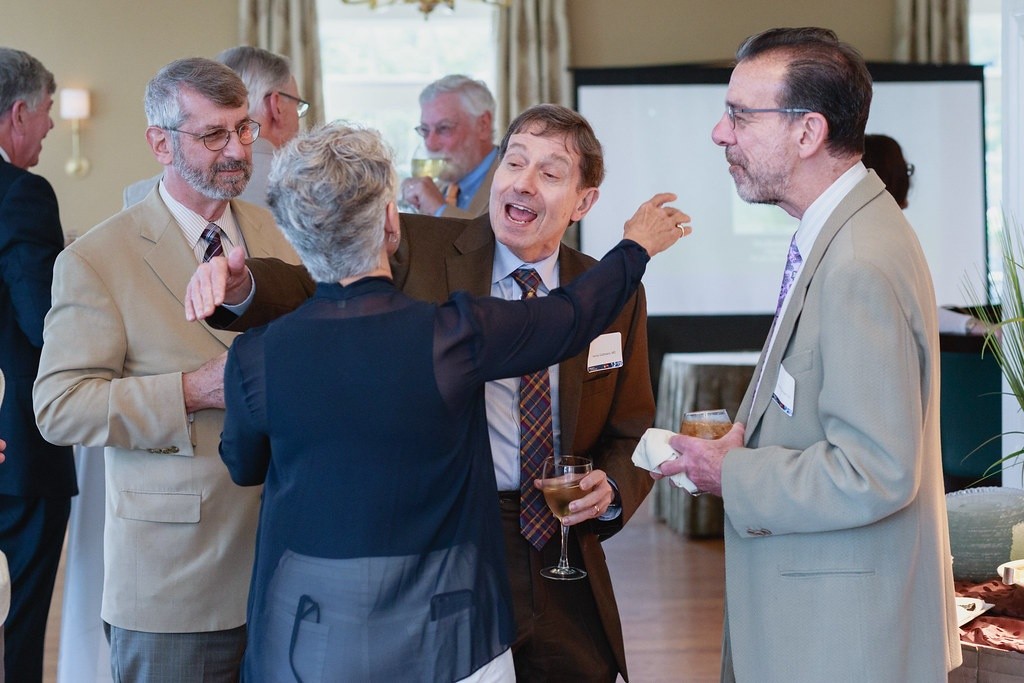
[751,231,803,414]
[510,267,560,552]
[201,224,226,263]
[445,183,459,208]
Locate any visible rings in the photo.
[676,224,684,238]
[409,184,413,188]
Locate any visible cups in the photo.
[680,408,733,443]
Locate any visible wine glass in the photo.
[397,143,453,216]
[540,455,593,581]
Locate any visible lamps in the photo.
[59,86,91,176]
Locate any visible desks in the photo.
[654,351,762,537]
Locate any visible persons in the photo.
[390,73,507,220]
[0,47,80,683]
[216,122,690,682]
[34,56,302,682]
[121,48,311,209]
[183,102,656,682]
[649,25,964,683]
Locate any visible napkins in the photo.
[632,427,712,497]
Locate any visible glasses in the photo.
[726,105,813,130]
[160,119,261,152]
[415,120,479,137]
[263,92,310,117]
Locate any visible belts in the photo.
[498,492,520,503]
